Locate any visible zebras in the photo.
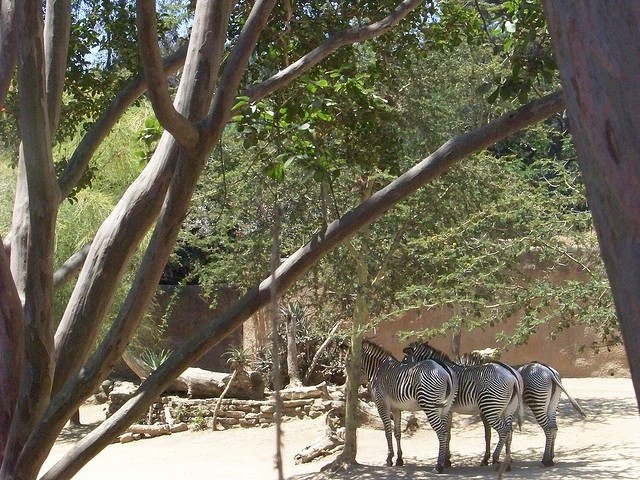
[402,341,524,472]
[460,353,587,468]
[345,339,459,474]
